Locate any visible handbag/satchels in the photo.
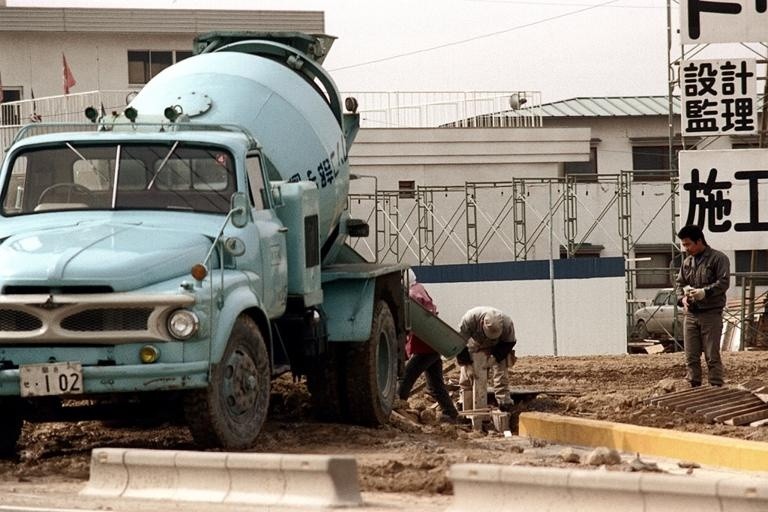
[506,350,518,368]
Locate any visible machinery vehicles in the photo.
[0,30,470,461]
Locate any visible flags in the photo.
[63,56,76,92]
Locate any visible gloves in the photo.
[683,285,706,302]
[459,365,474,386]
[482,354,496,370]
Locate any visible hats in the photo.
[483,311,504,339]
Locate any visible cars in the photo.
[634,288,684,342]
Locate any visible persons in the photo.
[458,306,517,412]
[676,225,730,387]
[399,268,458,419]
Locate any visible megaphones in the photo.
[510,94,526,109]
[126,92,138,105]
